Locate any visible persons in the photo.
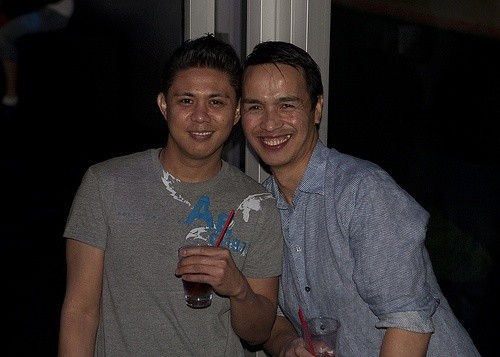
[240,41,484,357]
[57,34,282,357]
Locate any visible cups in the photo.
[178,243,214,309]
[302,316,341,357]
